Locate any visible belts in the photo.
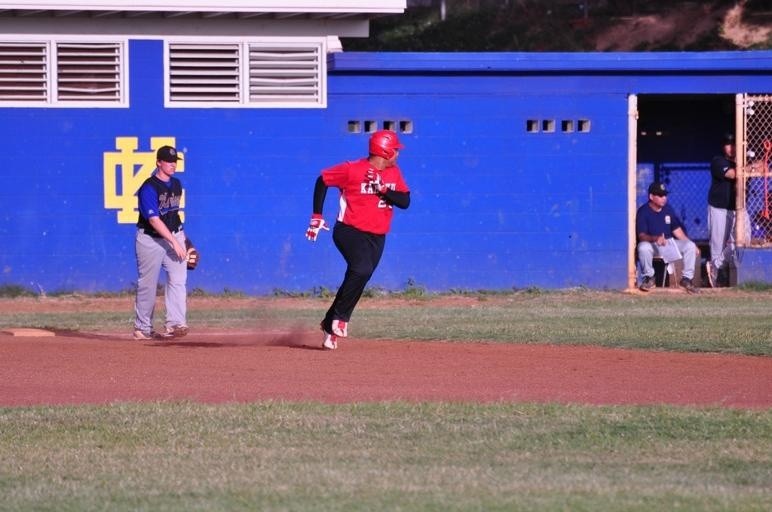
[142,226,184,237]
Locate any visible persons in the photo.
[635,183,702,296]
[132,145,190,343]
[705,130,768,287]
[304,130,411,351]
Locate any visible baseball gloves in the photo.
[185,238,199,270]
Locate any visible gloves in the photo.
[364,167,389,195]
[304,212,332,242]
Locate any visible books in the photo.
[654,237,683,264]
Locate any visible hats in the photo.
[647,182,669,195]
[720,133,737,145]
[156,144,183,163]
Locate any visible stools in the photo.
[652,255,678,288]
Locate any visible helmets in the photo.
[367,128,406,161]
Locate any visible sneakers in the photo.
[163,323,192,339]
[676,275,700,295]
[638,276,658,293]
[706,259,720,288]
[319,319,339,350]
[131,329,165,342]
[331,319,349,339]
